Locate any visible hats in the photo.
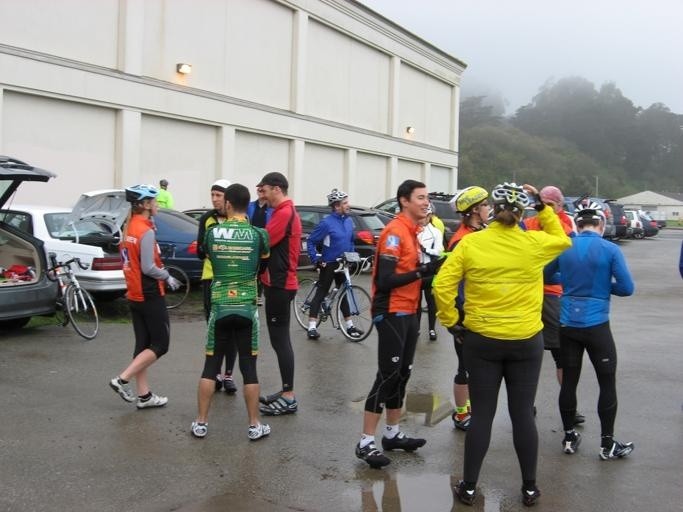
[211,179,231,191]
[255,172,287,188]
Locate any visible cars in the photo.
[0,156,59,332]
[183,207,314,267]
[0,188,134,311]
[154,209,210,291]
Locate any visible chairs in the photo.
[18,220,32,233]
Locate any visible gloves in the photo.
[165,275,182,289]
[317,259,326,269]
[417,256,447,277]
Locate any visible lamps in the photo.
[407,127,414,133]
[177,64,192,74]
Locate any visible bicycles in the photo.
[45,256,99,341]
[150,242,191,311]
[292,252,375,343]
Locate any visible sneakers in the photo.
[521,486,541,504]
[109,376,136,402]
[563,409,586,454]
[451,409,473,430]
[455,479,476,504]
[347,326,364,337]
[215,373,297,415]
[599,440,635,461]
[355,440,392,466]
[137,392,170,409]
[190,420,209,437]
[307,329,321,339]
[248,425,271,440]
[429,329,438,340]
[382,432,427,452]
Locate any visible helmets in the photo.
[326,188,347,206]
[125,183,159,203]
[449,181,608,221]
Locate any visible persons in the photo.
[247,182,274,305]
[356,181,443,468]
[544,198,635,460]
[196,179,238,395]
[190,183,272,441]
[108,184,185,410]
[305,190,364,339]
[522,187,585,422]
[156,179,174,210]
[256,172,303,415]
[439,186,491,430]
[433,183,573,505]
